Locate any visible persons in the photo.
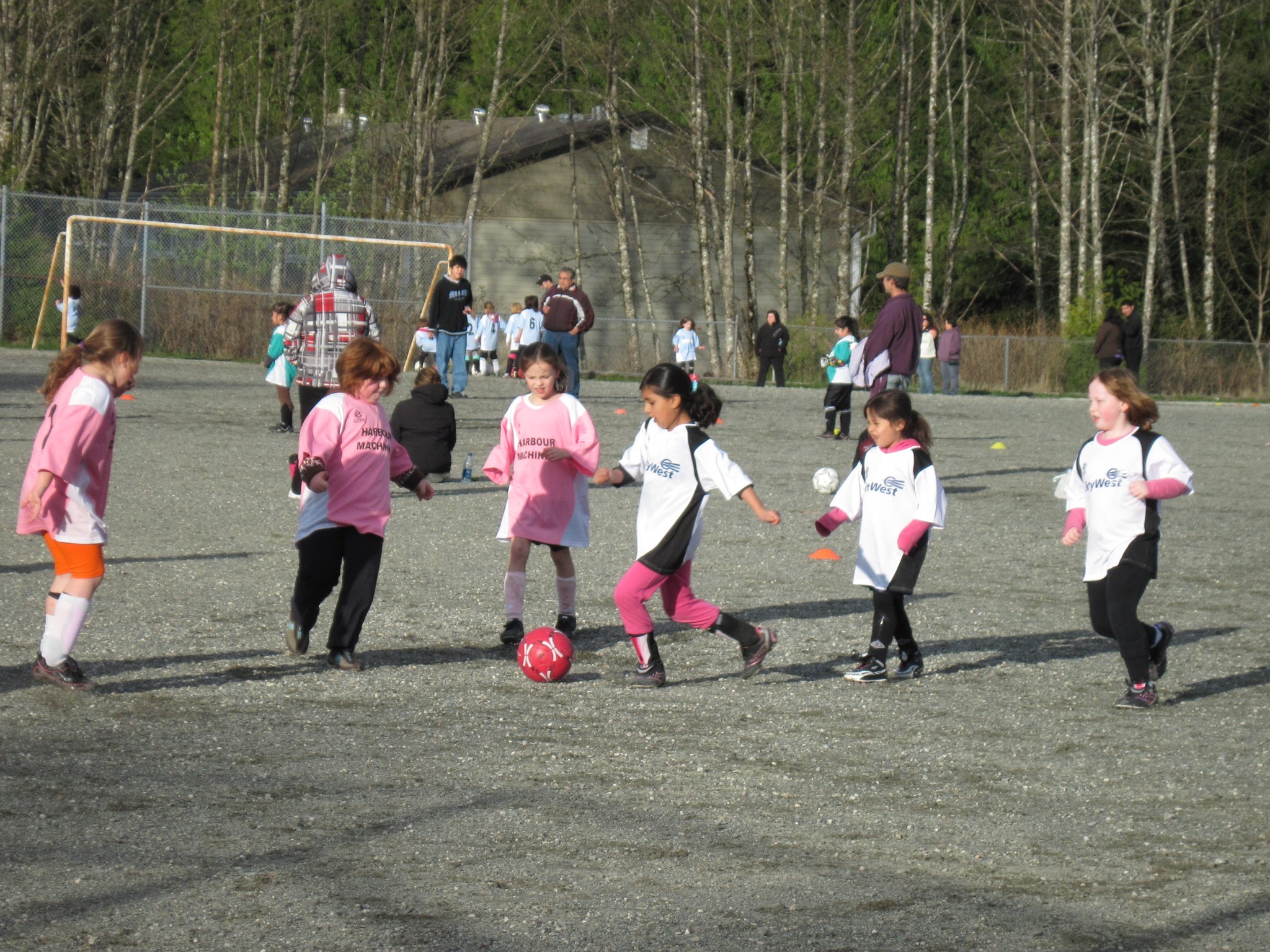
[504,302,523,378]
[863,262,923,391]
[815,389,947,682]
[464,308,481,377]
[1052,367,1195,710]
[415,318,436,370]
[815,315,863,441]
[282,254,380,427]
[474,301,507,377]
[754,310,790,387]
[261,301,297,434]
[1120,299,1143,382]
[1093,307,1125,370]
[285,335,434,671]
[16,318,145,691]
[539,267,595,399]
[514,295,546,378]
[536,274,566,368]
[55,279,84,345]
[389,367,456,483]
[594,363,781,687]
[481,342,600,643]
[937,317,962,395]
[428,254,473,399]
[672,316,705,375]
[916,313,939,394]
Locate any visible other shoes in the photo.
[451,392,468,398]
[816,432,835,439]
[426,472,450,483]
[833,433,850,440]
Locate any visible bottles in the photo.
[461,452,473,482]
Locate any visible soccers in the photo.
[511,366,525,379]
[471,364,476,376]
[812,467,839,494]
[517,626,573,683]
[415,360,428,372]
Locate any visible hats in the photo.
[536,274,551,284]
[876,262,911,278]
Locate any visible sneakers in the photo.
[555,614,577,638]
[619,660,665,687]
[31,652,98,692]
[895,652,924,678]
[1115,678,1158,709]
[742,626,777,681]
[269,426,293,433]
[844,650,888,682]
[328,648,363,671]
[1147,621,1174,681]
[286,620,309,656]
[500,618,524,643]
